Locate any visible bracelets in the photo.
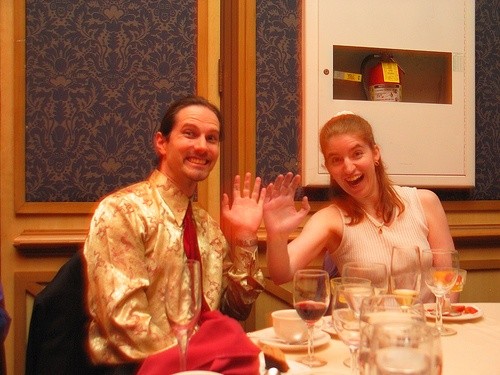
[236,235,259,247]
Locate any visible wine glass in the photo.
[342,262,389,367]
[165,259,202,371]
[294,270,331,367]
[420,249,459,335]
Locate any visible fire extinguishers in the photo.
[360,52,405,101]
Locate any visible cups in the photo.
[272,309,326,342]
[330,276,370,302]
[358,295,444,375]
[331,285,376,375]
[391,245,421,310]
[430,268,467,295]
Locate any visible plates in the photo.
[259,332,332,352]
[416,304,482,321]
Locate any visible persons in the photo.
[83,94,266,375]
[262,114,456,310]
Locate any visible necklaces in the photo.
[359,206,385,235]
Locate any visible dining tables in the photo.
[247,302,500,375]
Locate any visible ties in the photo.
[183,199,211,327]
